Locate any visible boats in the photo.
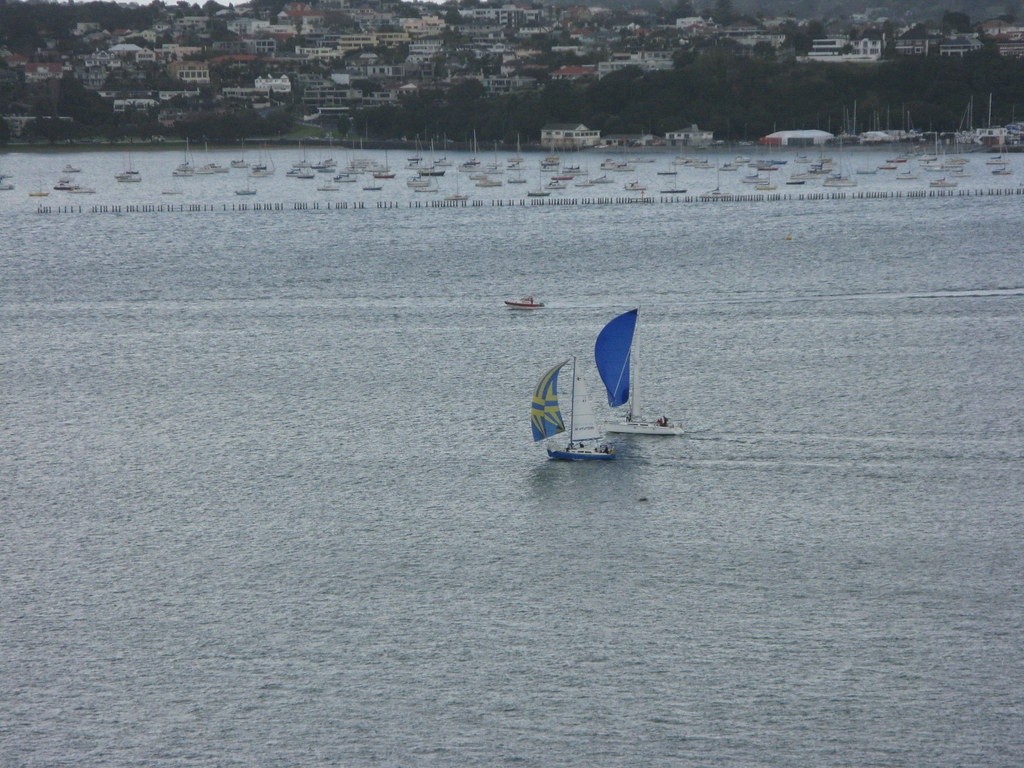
[504,293,546,309]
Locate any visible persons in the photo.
[579,441,584,448]
[600,445,609,453]
[657,416,668,427]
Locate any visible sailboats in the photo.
[528,354,617,461]
[593,302,685,437]
[0,125,1016,209]
